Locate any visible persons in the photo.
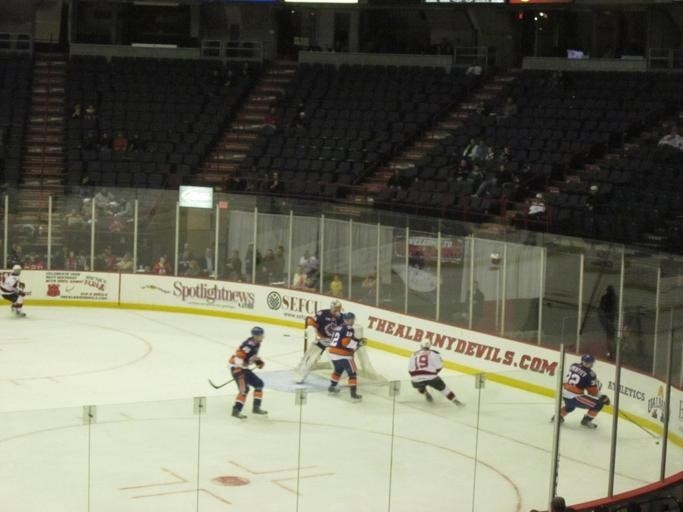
[657,127,683,155]
[226,327,269,419]
[296,301,342,384]
[549,353,610,431]
[598,282,617,358]
[327,311,369,400]
[408,343,463,408]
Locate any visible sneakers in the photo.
[581,419,597,428]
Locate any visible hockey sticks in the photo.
[610,401,663,440]
[208,365,260,389]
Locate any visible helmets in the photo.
[330,299,342,315]
[419,338,431,349]
[581,353,595,363]
[251,327,263,335]
[340,313,355,320]
[11,264,22,275]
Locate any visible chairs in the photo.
[64,49,683,259]
[1,49,33,186]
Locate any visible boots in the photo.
[328,386,340,393]
[16,310,26,317]
[351,389,362,399]
[232,405,247,419]
[253,401,267,414]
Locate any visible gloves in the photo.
[599,394,610,405]
[255,359,264,369]
[358,338,365,347]
